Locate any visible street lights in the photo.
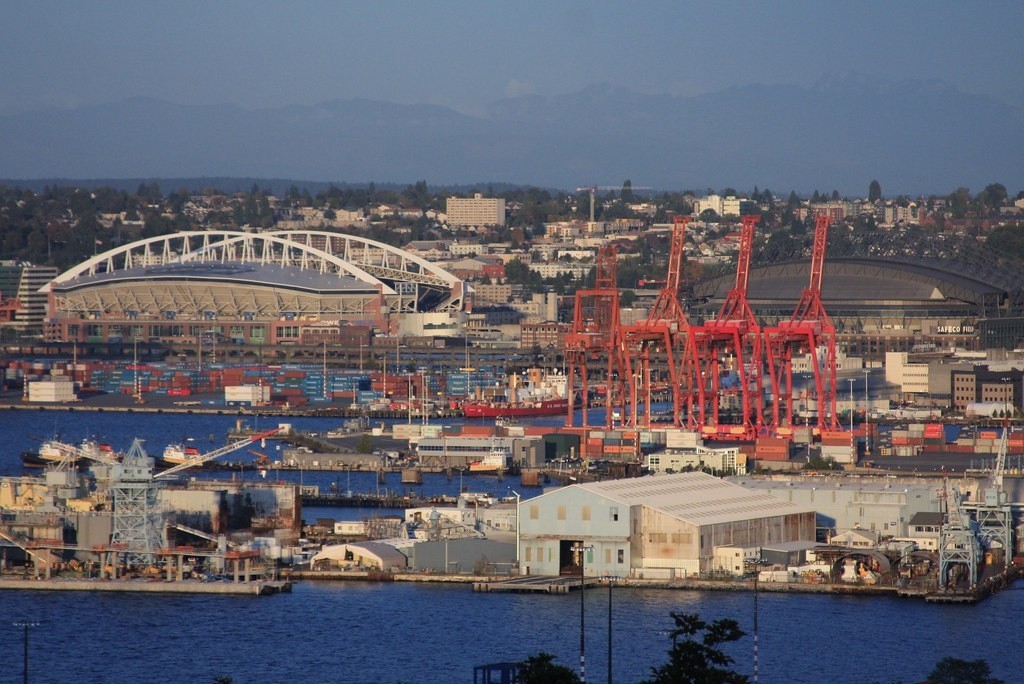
[743,558,774,684]
[1001,377,1011,428]
[407,366,431,427]
[598,574,623,684]
[863,370,872,455]
[570,544,597,684]
[802,375,812,464]
[632,374,642,458]
[645,368,654,453]
[846,378,858,470]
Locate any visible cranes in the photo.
[575,184,652,222]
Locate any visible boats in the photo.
[162,443,203,467]
[22,427,127,466]
